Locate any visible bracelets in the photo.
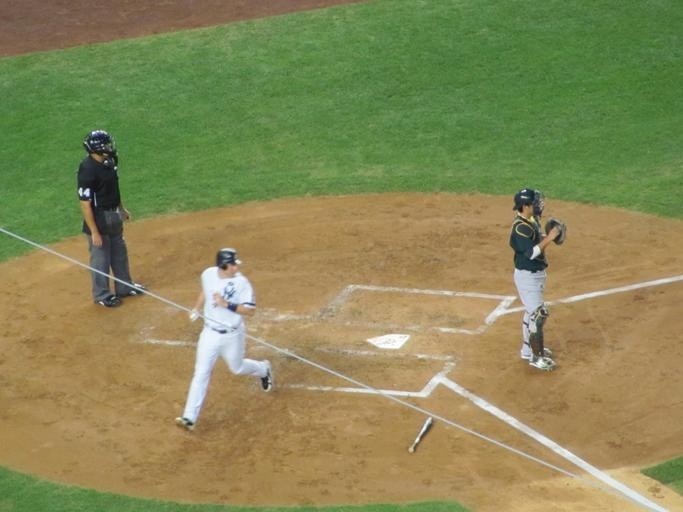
[227,301,238,314]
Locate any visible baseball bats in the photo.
[408,417,433,453]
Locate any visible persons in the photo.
[509,189,560,371]
[176,248,271,430]
[76,130,147,308]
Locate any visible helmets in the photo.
[82,130,119,158]
[511,188,545,218]
[215,247,242,269]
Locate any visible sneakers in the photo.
[175,416,194,432]
[529,354,556,371]
[520,346,552,361]
[94,294,121,308]
[116,282,147,298]
[259,359,273,394]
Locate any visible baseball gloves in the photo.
[546,218,566,244]
